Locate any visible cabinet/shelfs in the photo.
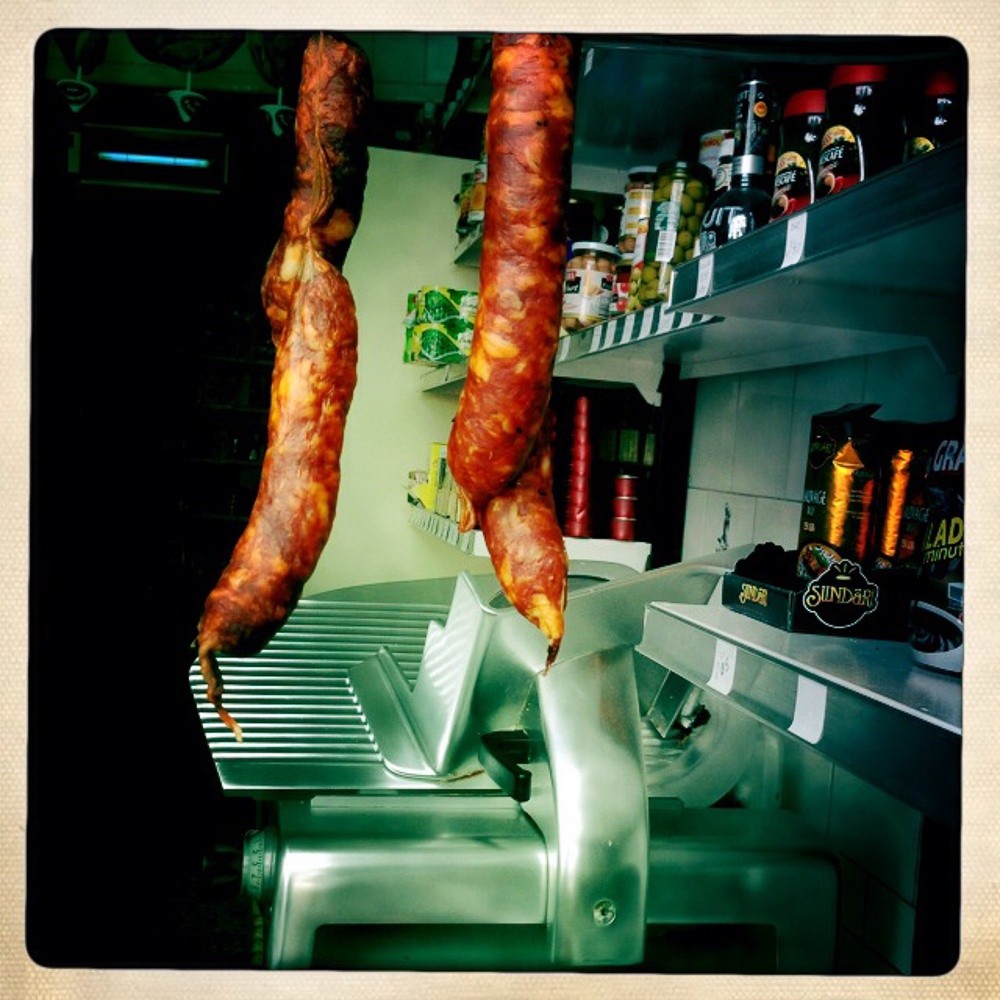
[413,61,964,977]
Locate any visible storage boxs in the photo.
[799,407,964,577]
[719,557,913,635]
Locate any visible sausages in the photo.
[195,36,370,742]
[453,33,570,675]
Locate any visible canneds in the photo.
[611,474,639,540]
[557,159,713,332]
[727,79,778,191]
